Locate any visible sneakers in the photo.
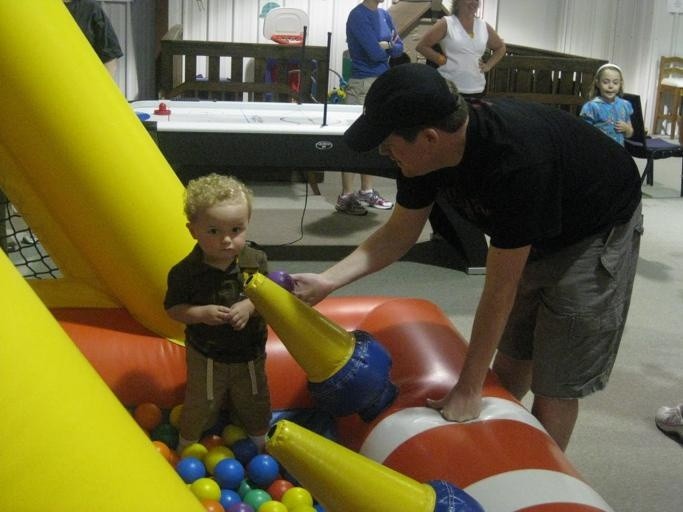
[655,402,682,441]
[334,192,368,216]
[358,188,393,210]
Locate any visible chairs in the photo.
[649,54,683,144]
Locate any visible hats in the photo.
[343,63,462,154]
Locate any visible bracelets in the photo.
[438,55,445,65]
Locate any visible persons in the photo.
[387,40,393,49]
[334,0,404,215]
[161,169,274,458]
[414,0,506,100]
[300,62,643,454]
[579,62,635,148]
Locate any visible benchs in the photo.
[548,75,682,198]
